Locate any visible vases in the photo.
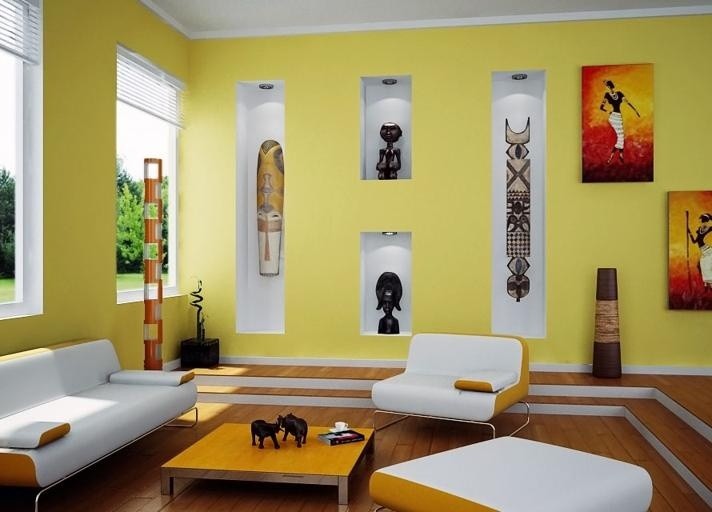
[593,268,621,378]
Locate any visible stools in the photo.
[369,436,653,512]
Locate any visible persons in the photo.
[599,80,640,164]
[376,284,401,333]
[688,213,712,290]
[376,123,402,179]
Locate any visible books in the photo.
[318,430,364,446]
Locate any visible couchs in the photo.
[0,337,198,512]
[371,332,530,438]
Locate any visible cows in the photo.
[278,413,308,447]
[251,419,284,449]
